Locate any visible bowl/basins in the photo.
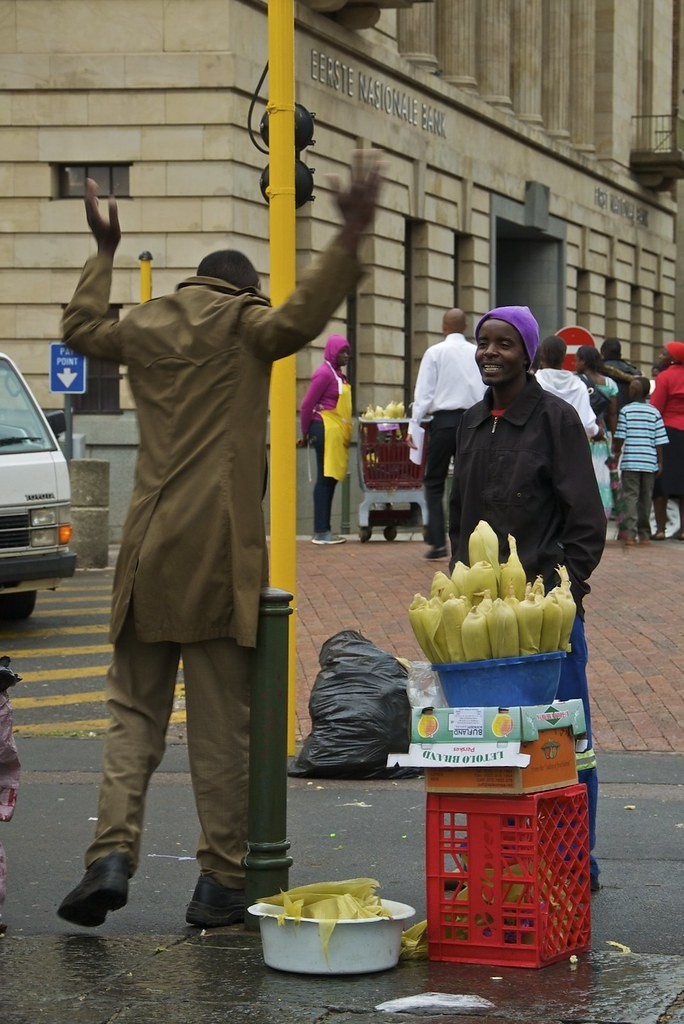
[431,648,567,706]
[247,899,416,974]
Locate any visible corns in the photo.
[361,400,405,419]
[408,520,576,665]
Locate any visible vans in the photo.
[0,352,77,626]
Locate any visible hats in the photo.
[476,306,538,372]
[666,341,684,364]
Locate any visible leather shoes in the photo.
[185,875,246,925]
[57,852,133,927]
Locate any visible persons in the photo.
[298,335,352,544]
[613,377,670,544]
[600,338,642,411]
[56,148,389,928]
[650,342,684,541]
[449,306,607,894]
[533,336,607,443]
[573,344,619,508]
[405,308,489,563]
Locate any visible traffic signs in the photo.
[50,344,86,395]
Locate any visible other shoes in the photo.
[650,531,665,541]
[640,539,650,545]
[426,546,446,558]
[443,868,468,889]
[625,536,637,544]
[673,530,684,540]
[567,870,599,891]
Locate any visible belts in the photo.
[435,409,466,415]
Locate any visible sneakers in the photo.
[311,531,346,545]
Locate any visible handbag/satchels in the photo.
[576,372,610,416]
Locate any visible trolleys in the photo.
[355,419,431,543]
